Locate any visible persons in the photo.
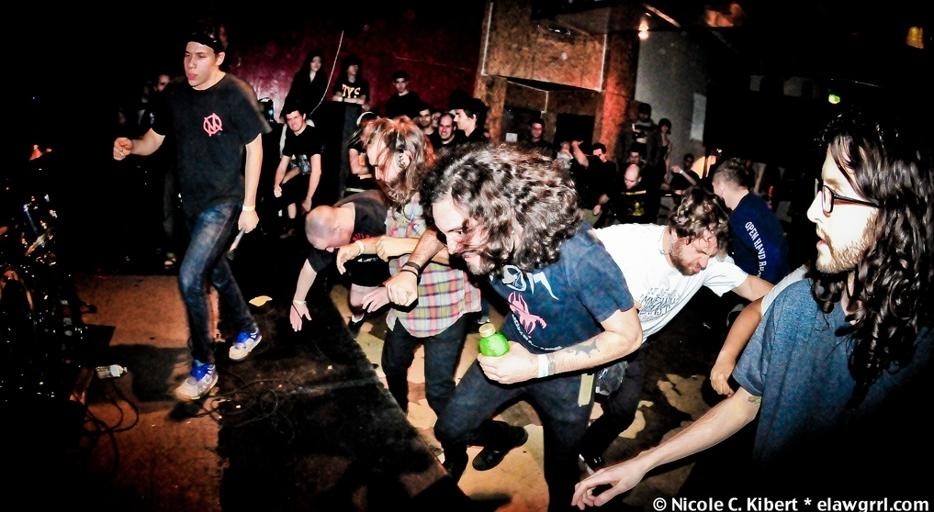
[106,17,932,511]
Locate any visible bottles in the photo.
[96,364,127,380]
[479,323,510,357]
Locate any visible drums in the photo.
[5,194,59,256]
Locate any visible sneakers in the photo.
[347,314,367,339]
[442,453,468,484]
[579,450,604,475]
[472,427,529,470]
[173,359,219,402]
[228,326,263,362]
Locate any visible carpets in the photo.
[218,290,474,511]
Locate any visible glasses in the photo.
[814,178,880,213]
